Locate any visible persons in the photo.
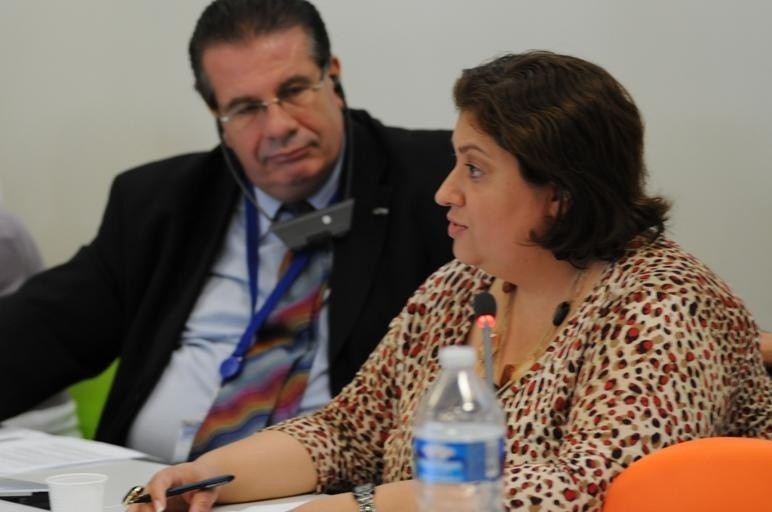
[0,0,456,463]
[126,52,771,512]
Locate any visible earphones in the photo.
[329,73,347,99]
[216,116,225,140]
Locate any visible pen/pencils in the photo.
[133,475,234,503]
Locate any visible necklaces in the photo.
[473,263,587,398]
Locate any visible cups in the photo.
[44,471,108,511]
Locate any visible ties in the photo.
[188,198,330,462]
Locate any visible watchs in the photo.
[353,482,375,512]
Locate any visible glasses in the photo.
[218,61,330,131]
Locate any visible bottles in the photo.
[410,346,510,511]
